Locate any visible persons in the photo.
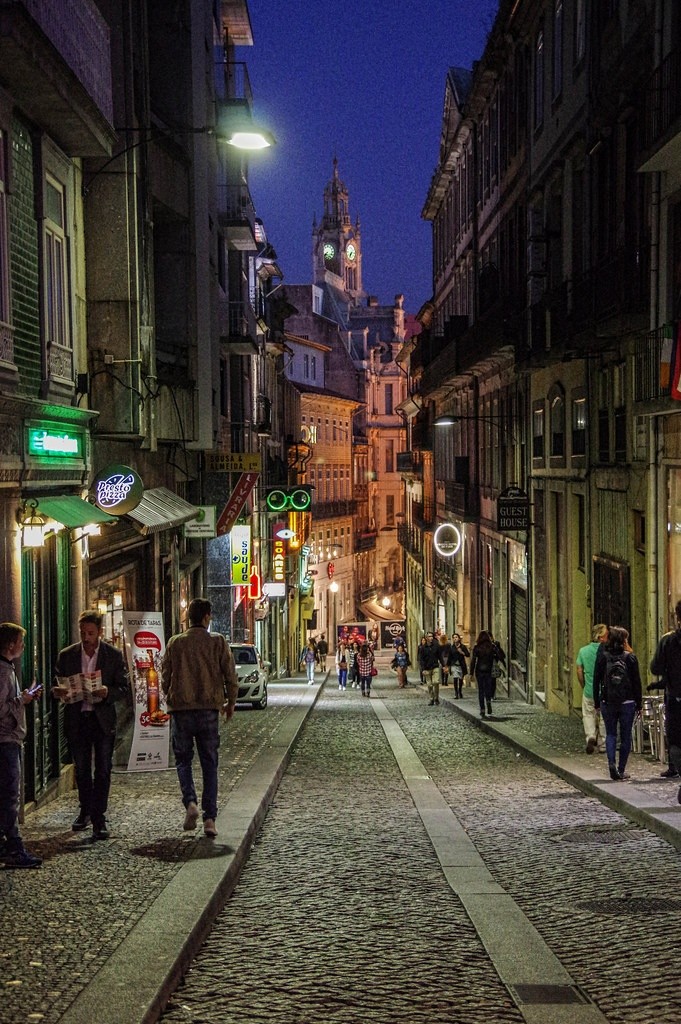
[335,641,377,696]
[339,623,379,650]
[159,598,239,836]
[576,624,611,754]
[317,634,329,672]
[49,611,130,838]
[593,626,644,779]
[299,638,320,685]
[0,622,45,868]
[649,601,681,805]
[390,630,506,716]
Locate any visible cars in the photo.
[223,643,272,709]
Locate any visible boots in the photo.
[445,674,447,685]
[454,678,458,699]
[459,679,463,698]
[441,669,444,685]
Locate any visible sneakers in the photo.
[72,812,93,832]
[184,801,198,830]
[0,835,42,869]
[203,818,218,836]
[90,814,109,838]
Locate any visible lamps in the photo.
[311,544,342,551]
[380,527,408,531]
[81,123,277,195]
[82,494,103,536]
[432,415,517,441]
[19,496,46,548]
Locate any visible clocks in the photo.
[346,244,356,260]
[323,243,336,260]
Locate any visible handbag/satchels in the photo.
[371,669,377,676]
[339,662,347,670]
[491,644,501,677]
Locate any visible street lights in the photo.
[330,582,338,653]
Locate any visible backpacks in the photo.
[603,649,632,703]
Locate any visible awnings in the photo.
[21,495,119,529]
[121,487,201,537]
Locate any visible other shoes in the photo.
[338,675,370,696]
[586,737,595,754]
[609,766,620,780]
[434,699,439,705]
[428,699,433,705]
[487,702,492,714]
[480,706,485,717]
[598,745,607,753]
[619,771,630,778]
[661,768,677,776]
